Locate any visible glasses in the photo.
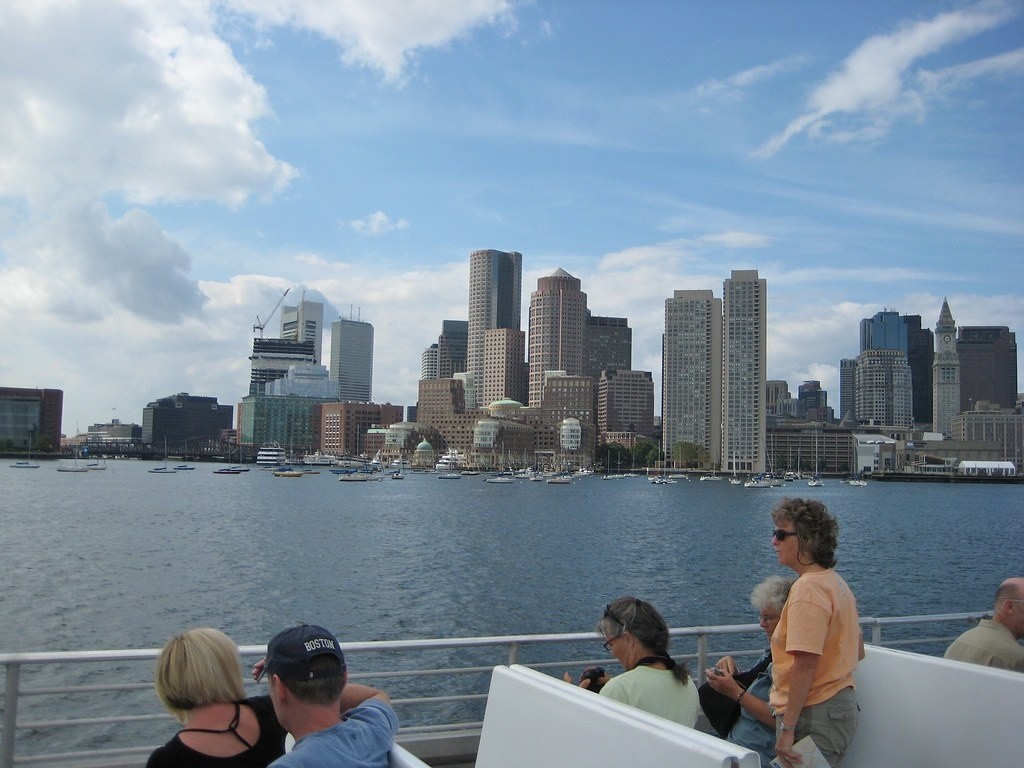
[1003,599,1024,604]
[602,635,620,651]
[757,614,777,621]
[772,529,798,541]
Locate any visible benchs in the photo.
[388,645,1024,768]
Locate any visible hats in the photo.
[257,623,346,686]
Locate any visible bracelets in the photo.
[737,689,745,702]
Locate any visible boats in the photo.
[85,457,108,471]
[255,443,286,467]
[272,448,593,484]
[840,470,868,488]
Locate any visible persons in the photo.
[944,577,1024,672]
[145,627,288,768]
[563,596,699,729]
[698,575,798,768]
[770,496,865,768]
[252,624,400,768]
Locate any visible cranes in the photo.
[251,288,291,338]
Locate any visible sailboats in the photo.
[9,433,40,469]
[212,442,252,474]
[148,436,177,473]
[599,432,824,488]
[56,429,90,473]
[172,441,195,471]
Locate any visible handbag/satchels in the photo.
[698,652,772,740]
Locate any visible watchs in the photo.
[780,722,796,730]
